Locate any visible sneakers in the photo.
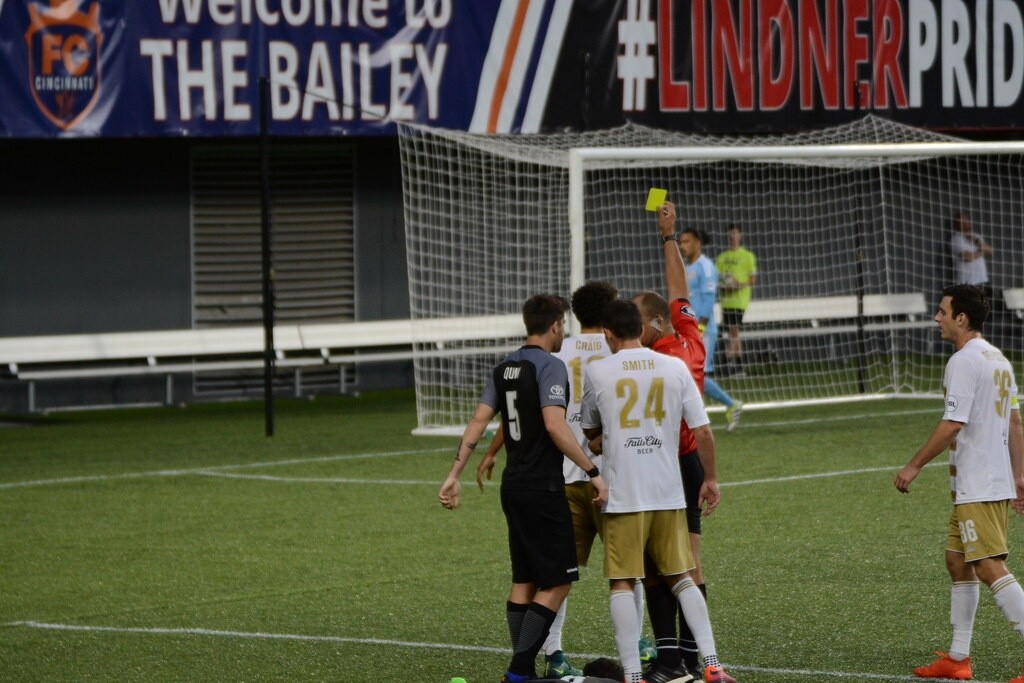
[913,651,971,680]
[725,398,741,433]
[503,648,733,683]
[645,664,693,683]
[544,650,583,678]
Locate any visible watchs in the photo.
[662,232,678,244]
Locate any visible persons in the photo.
[715,223,758,379]
[439,200,738,683]
[951,212,994,294]
[893,282,1024,683]
[679,228,744,432]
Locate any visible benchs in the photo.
[0,292,928,413]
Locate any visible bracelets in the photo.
[584,465,600,478]
